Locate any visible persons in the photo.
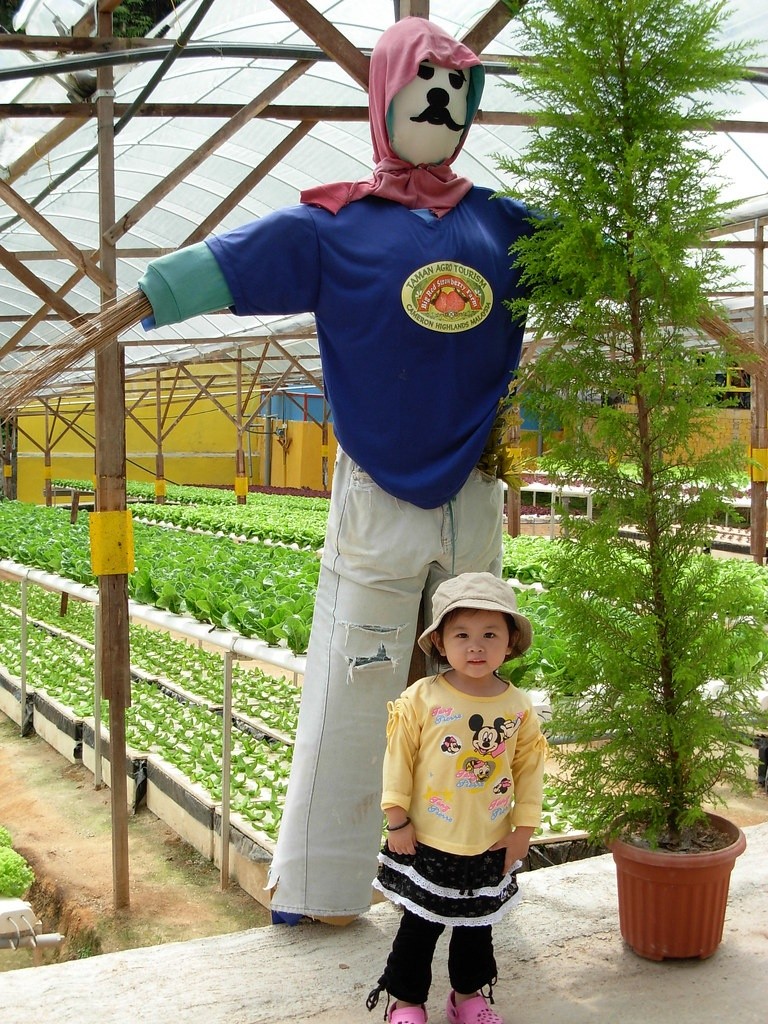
[141,16,611,928]
[369,572,547,1024]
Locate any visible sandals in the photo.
[388,999,429,1024]
[446,988,503,1024]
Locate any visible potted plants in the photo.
[503,0,768,965]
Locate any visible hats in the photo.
[416,575,533,665]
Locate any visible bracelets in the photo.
[385,815,411,832]
